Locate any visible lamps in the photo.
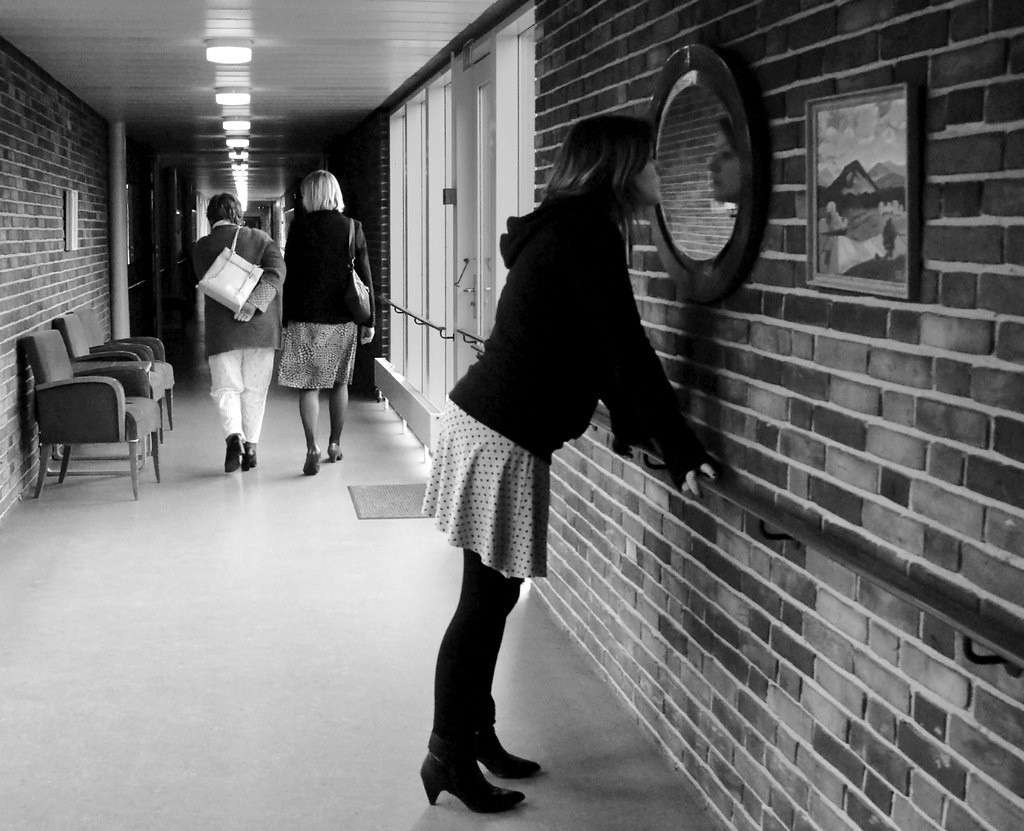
[215,86,250,105]
[205,38,253,64]
[226,135,250,147]
[228,147,249,171]
[223,116,251,130]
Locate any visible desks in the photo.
[42,361,152,476]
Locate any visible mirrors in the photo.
[646,44,772,302]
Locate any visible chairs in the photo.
[22,329,161,501]
[68,308,174,431]
[51,313,163,454]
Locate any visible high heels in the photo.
[421,732,526,813]
[328,443,343,463]
[241,443,256,471]
[225,433,245,472]
[303,444,321,475]
[475,725,541,780]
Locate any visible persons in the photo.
[273,169,375,475]
[418,114,718,813]
[706,115,743,208]
[190,192,288,473]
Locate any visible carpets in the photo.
[347,484,427,519]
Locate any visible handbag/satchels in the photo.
[198,225,264,314]
[348,217,371,325]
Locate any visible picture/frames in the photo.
[805,81,921,300]
[66,190,78,250]
